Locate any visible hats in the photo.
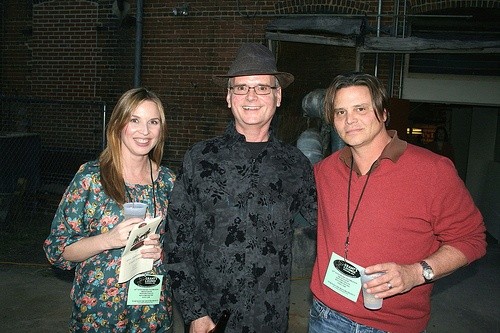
[212,44,295,89]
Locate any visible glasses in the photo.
[229,84,276,95]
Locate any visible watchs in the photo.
[416,259,434,284]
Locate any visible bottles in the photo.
[208,311,230,333]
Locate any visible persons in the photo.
[166,41,320,333]
[430,126,456,170]
[41,84,179,332]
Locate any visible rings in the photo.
[302,69,487,333]
[384,280,394,291]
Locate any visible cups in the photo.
[360,269,385,309]
[123,203,147,223]
